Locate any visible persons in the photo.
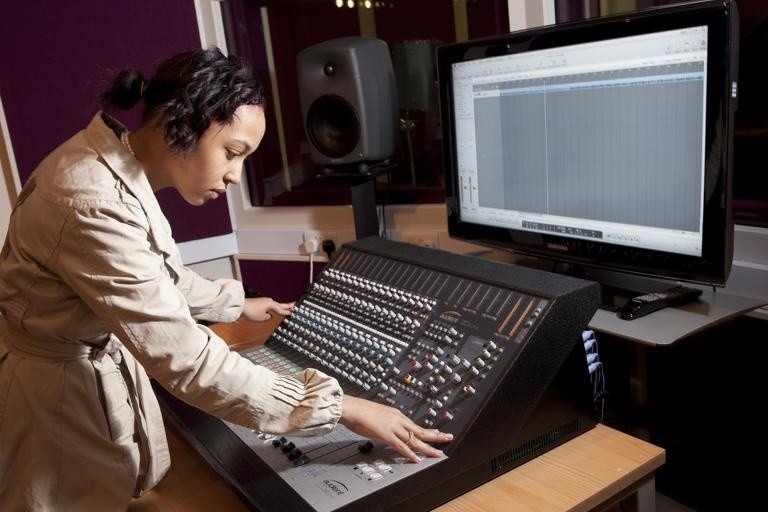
[0,44,455,512]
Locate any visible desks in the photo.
[589,286,768,511]
[145,300,665,512]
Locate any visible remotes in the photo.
[620,285,704,320]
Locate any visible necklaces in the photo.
[119,129,138,163]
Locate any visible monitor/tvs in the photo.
[434,0,741,313]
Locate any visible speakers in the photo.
[295,35,401,174]
[393,37,441,113]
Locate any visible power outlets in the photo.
[303,230,339,255]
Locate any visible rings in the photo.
[404,430,414,446]
